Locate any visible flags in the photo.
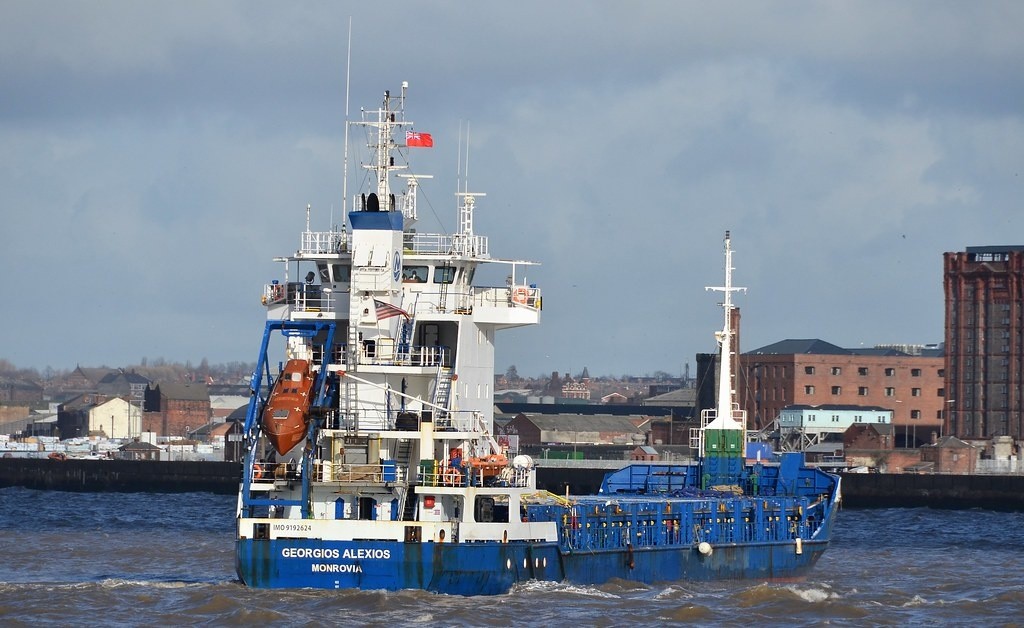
[406,131,433,148]
[374,299,411,324]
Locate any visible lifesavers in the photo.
[445,468,460,483]
[252,464,261,478]
[514,288,528,303]
[273,286,283,299]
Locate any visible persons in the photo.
[409,269,421,281]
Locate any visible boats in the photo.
[228,17,843,595]
